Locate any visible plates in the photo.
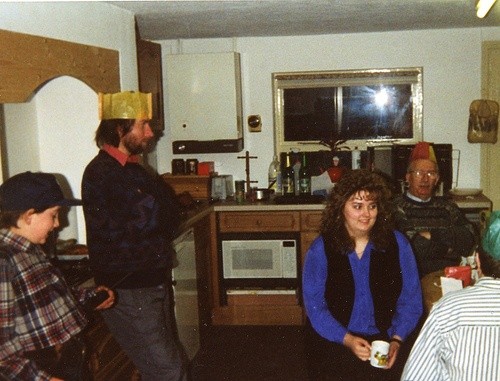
[449,187,483,195]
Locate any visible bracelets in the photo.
[388,338,402,345]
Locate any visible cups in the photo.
[366,341,390,368]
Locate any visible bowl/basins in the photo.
[255,189,274,200]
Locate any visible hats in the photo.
[481,211,500,261]
[409,142,436,164]
[0,170,85,212]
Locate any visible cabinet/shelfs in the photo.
[196,211,325,335]
[136,38,165,132]
[162,51,243,154]
[86,314,144,381]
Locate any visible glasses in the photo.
[408,168,438,179]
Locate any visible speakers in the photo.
[391,144,453,191]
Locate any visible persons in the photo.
[385,142,491,280]
[0,171,115,381]
[400,211,500,381]
[81,89,196,381]
[301,169,423,381]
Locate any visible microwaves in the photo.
[221,239,297,279]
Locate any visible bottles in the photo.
[298,152,311,194]
[172,159,185,176]
[268,155,282,199]
[186,158,198,176]
[351,146,361,170]
[282,155,295,194]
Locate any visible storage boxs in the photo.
[162,161,215,202]
